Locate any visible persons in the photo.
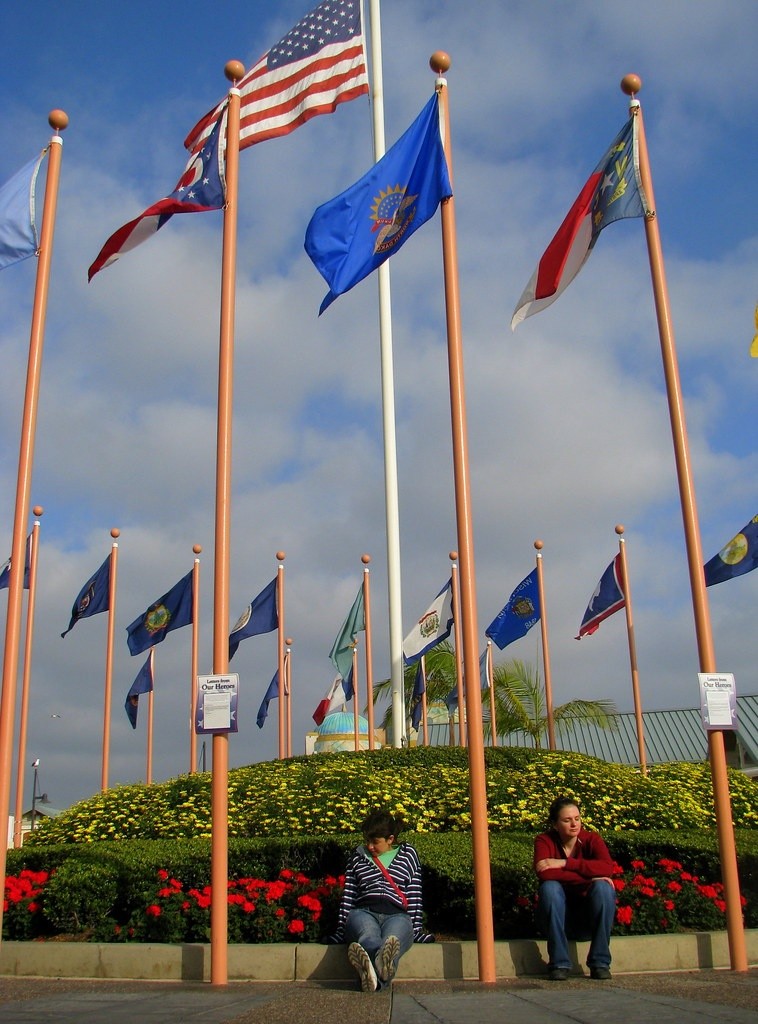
[534,796,615,980]
[329,811,435,993]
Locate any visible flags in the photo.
[303,91,452,318]
[485,565,541,650]
[256,656,289,730]
[312,664,355,726]
[574,551,625,640]
[184,0,368,157]
[88,102,229,283]
[61,552,112,639]
[704,513,758,587]
[126,567,194,656]
[0,148,47,270]
[409,660,425,732]
[0,533,32,590]
[443,648,490,715]
[124,651,153,730]
[511,106,651,332]
[403,575,454,666]
[229,575,279,662]
[329,579,365,682]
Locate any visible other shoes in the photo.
[550,968,570,979]
[590,968,612,980]
[375,935,399,982]
[348,942,377,993]
